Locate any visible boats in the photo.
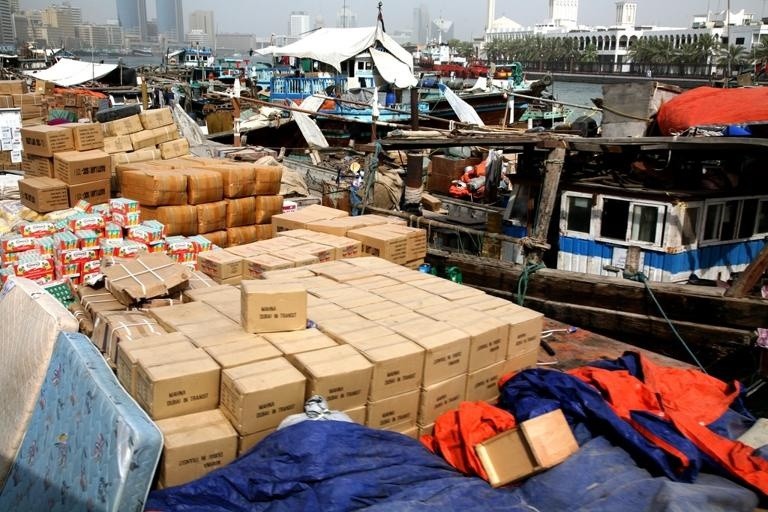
[161,0,768,370]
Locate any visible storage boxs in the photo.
[53,203,545,491]
[0,73,196,214]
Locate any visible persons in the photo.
[500,171,513,208]
